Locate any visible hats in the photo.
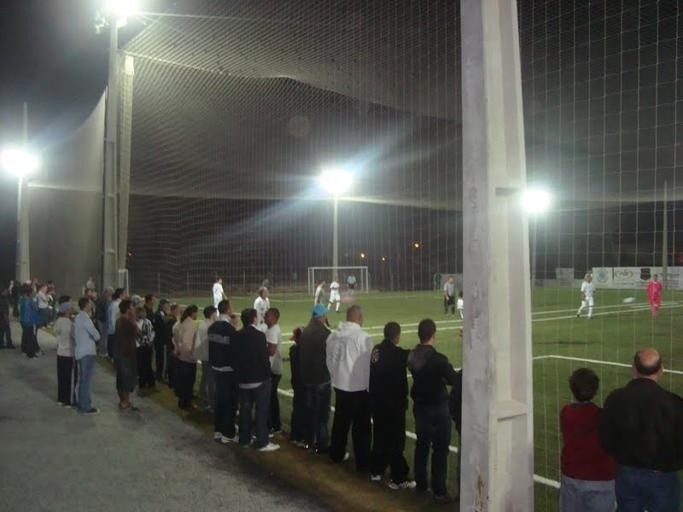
[57,302,72,313]
[129,294,145,305]
[308,303,330,318]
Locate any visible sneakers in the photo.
[368,472,384,483]
[269,426,281,432]
[328,451,350,464]
[116,402,141,412]
[220,435,240,446]
[62,403,72,410]
[77,406,97,414]
[6,344,17,350]
[211,430,222,441]
[432,492,458,506]
[257,441,281,453]
[384,478,417,491]
[238,435,255,449]
[0,344,7,349]
[288,438,299,444]
[415,488,433,497]
[54,400,62,405]
[296,441,305,448]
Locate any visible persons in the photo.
[171,275,285,451]
[597,347,683,512]
[444,276,456,316]
[289,304,373,472]
[314,274,357,313]
[404,318,463,501]
[367,320,416,489]
[576,273,596,319]
[1,275,170,414]
[645,273,662,315]
[558,367,618,512]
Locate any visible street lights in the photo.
[2,145,40,283]
[312,166,359,266]
[525,185,553,283]
[96,0,148,288]
